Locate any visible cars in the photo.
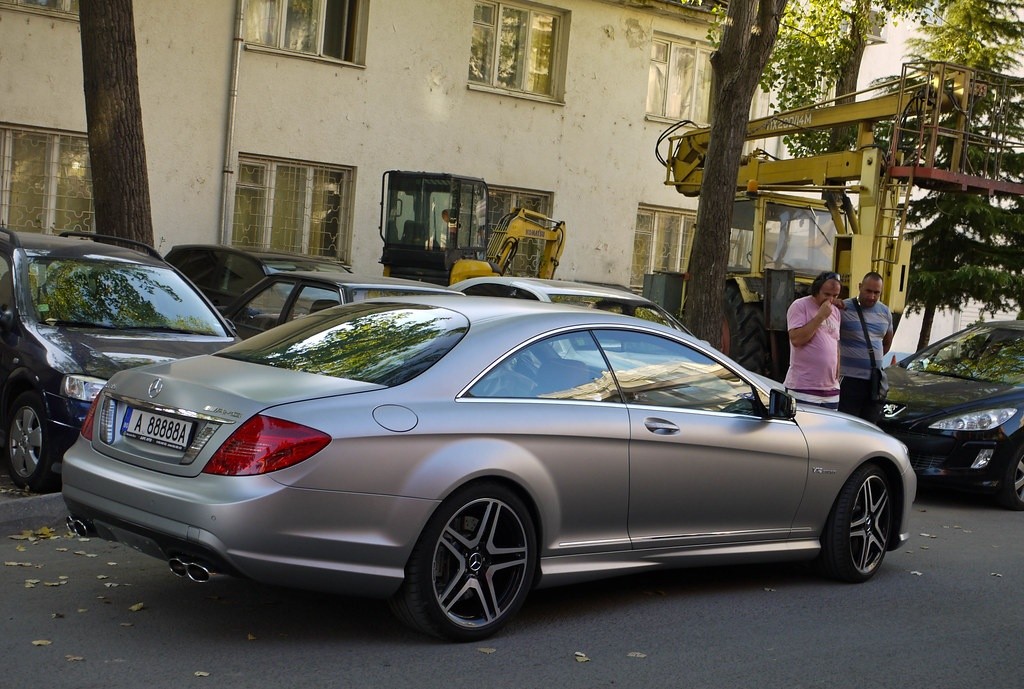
[163,243,355,317]
[58,294,919,642]
[877,321,1023,510]
[222,269,468,344]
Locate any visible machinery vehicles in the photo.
[379,168,566,288]
[641,60,1022,377]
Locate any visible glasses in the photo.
[826,274,841,281]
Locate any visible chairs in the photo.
[530,358,593,397]
[308,300,340,314]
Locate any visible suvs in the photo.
[0,226,244,493]
[446,275,723,374]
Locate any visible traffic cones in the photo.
[891,355,896,365]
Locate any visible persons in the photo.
[829,270,893,424]
[781,269,843,409]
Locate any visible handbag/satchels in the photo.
[872,368,889,402]
